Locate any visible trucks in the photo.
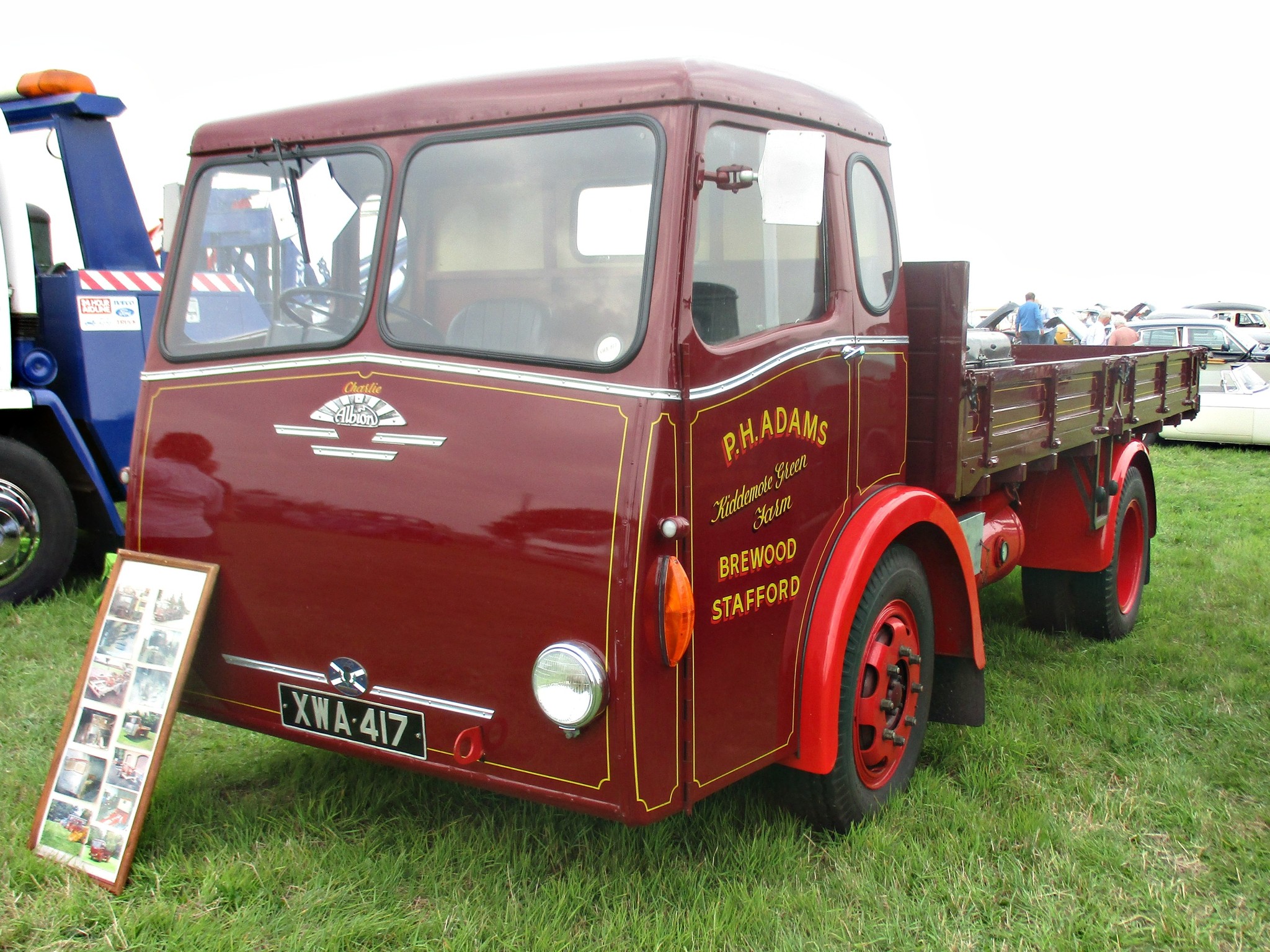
[0,65,405,607]
[123,58,1210,835]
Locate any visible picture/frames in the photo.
[28,549,219,895]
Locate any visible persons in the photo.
[1015,292,1057,345]
[1080,311,1111,346]
[1107,314,1139,346]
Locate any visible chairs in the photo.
[444,298,554,360]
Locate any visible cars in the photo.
[966,300,1116,369]
[1043,318,1270,447]
[1076,299,1270,344]
